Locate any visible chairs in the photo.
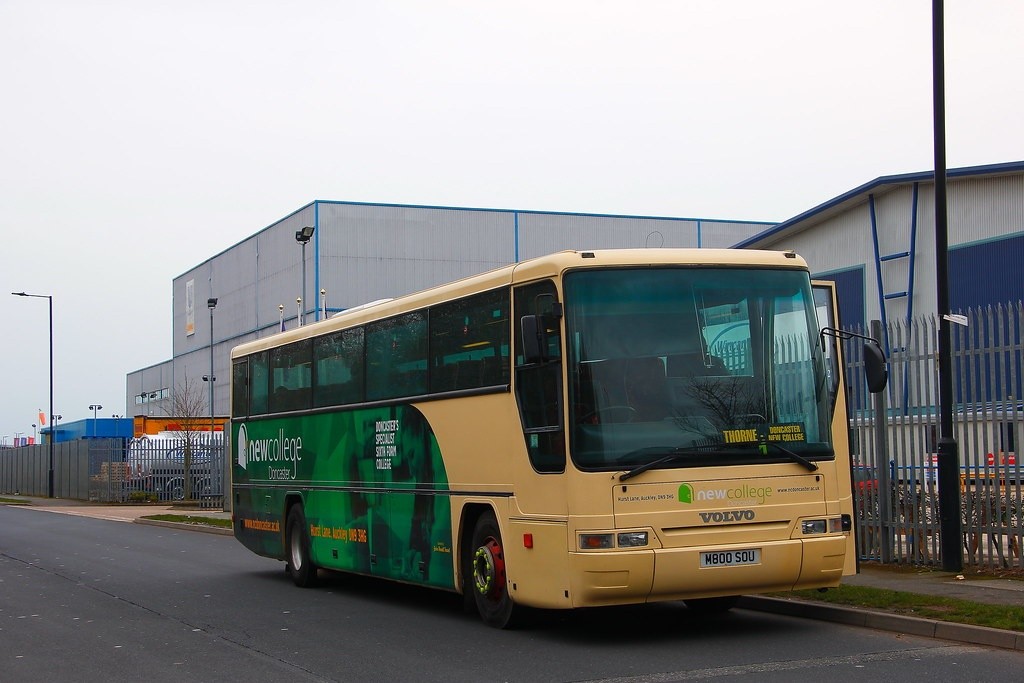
[252,354,733,424]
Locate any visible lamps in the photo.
[141,391,150,399]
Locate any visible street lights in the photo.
[294,226,316,326]
[11,292,54,499]
[202,297,219,441]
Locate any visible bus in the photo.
[225,242,892,631]
